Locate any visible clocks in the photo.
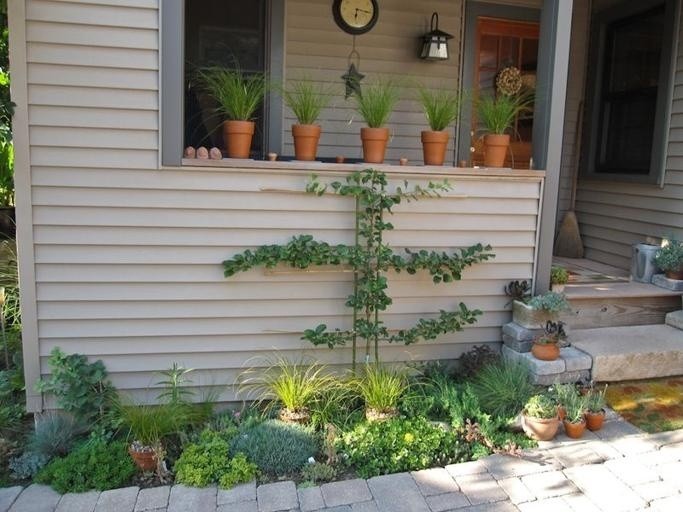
[332,0,379,99]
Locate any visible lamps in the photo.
[415,12,454,61]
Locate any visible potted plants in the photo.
[277,80,341,161]
[192,62,280,158]
[342,362,433,424]
[104,388,190,470]
[555,383,579,422]
[413,78,465,166]
[651,239,683,280]
[521,395,560,442]
[236,348,345,424]
[503,279,569,330]
[476,358,532,432]
[344,74,403,164]
[551,267,569,293]
[530,321,566,362]
[472,89,547,168]
[564,396,586,438]
[585,384,609,431]
